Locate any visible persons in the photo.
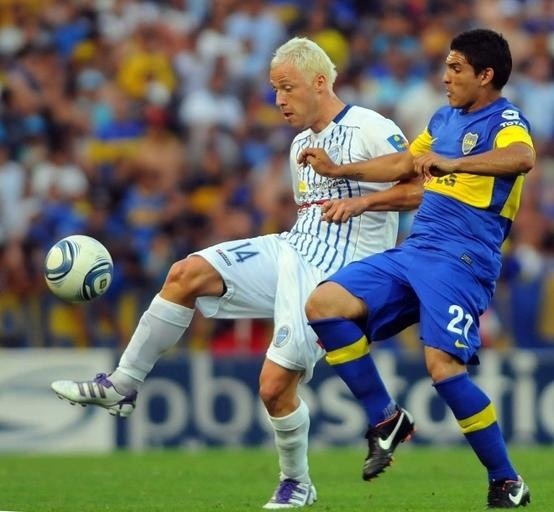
[49,36,425,509]
[295,28,530,509]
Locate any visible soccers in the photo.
[45,234,114,304]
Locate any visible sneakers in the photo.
[363,408,414,480]
[50,374,138,417]
[263,476,316,509]
[488,476,530,507]
